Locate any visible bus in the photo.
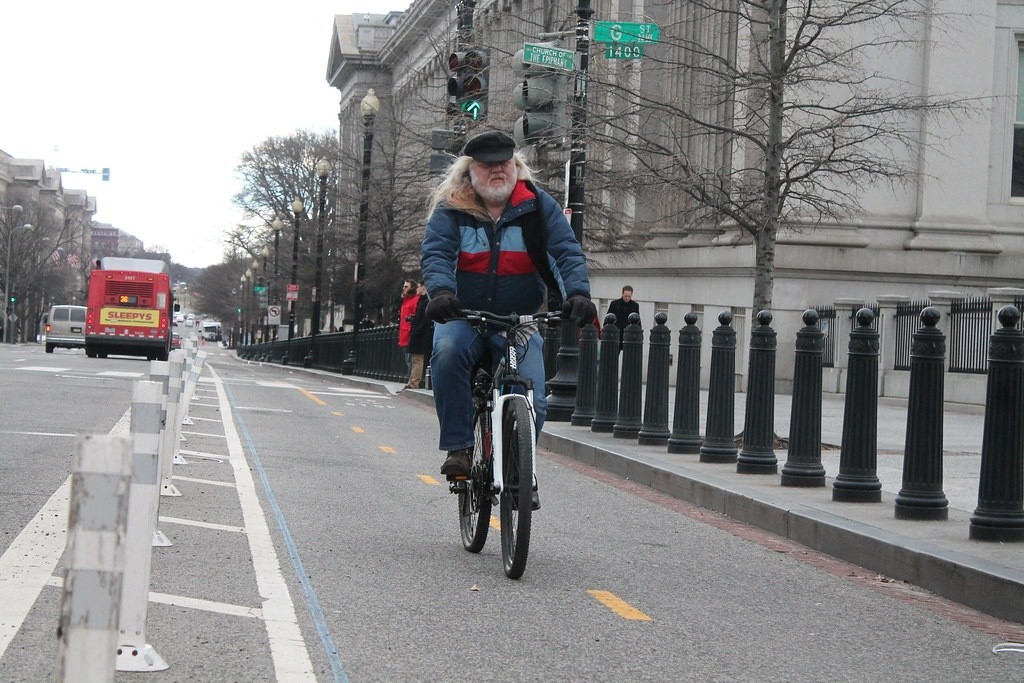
[85,257,181,362]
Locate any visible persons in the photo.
[420,132,597,515]
[397,279,432,394]
[606,286,639,350]
[360,314,374,329]
[331,326,343,333]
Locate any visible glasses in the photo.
[416,286,422,288]
[403,286,408,288]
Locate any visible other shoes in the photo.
[440,449,472,481]
[396,384,411,393]
[510,489,541,510]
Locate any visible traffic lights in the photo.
[446,52,471,115]
[519,39,567,146]
[508,47,541,150]
[461,49,490,122]
[10,290,16,303]
[429,127,452,174]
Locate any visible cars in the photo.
[173,313,223,342]
[172,333,183,351]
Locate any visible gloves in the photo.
[563,294,597,328]
[428,290,462,324]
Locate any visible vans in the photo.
[42,304,88,353]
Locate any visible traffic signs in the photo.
[521,41,579,73]
[590,18,661,45]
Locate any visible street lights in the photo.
[259,245,269,362]
[264,216,284,363]
[235,259,260,361]
[280,196,305,366]
[2,223,33,342]
[300,154,332,367]
[340,88,380,374]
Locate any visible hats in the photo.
[464,131,516,162]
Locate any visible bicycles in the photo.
[430,305,579,582]
